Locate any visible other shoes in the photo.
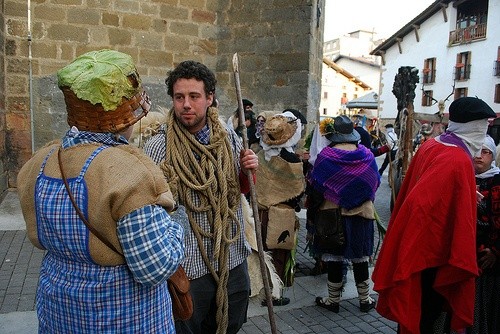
[315,283,344,314]
[356,281,376,313]
[261,296,291,306]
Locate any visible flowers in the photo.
[320,118,336,134]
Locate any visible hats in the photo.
[260,110,302,151]
[448,96,498,124]
[282,108,307,125]
[241,98,253,107]
[385,123,394,129]
[319,114,362,142]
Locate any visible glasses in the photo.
[481,150,491,155]
[256,120,265,122]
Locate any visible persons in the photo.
[371,96,497,334]
[248,107,315,307]
[227,98,259,129]
[145,60,259,334]
[376,123,398,175]
[471,133,500,334]
[305,115,378,313]
[233,112,258,204]
[18,47,190,334]
